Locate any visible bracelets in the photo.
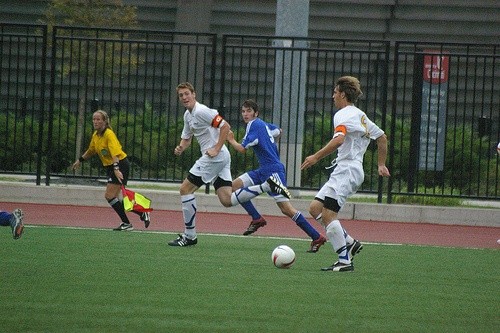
[113,167,119,170]
[79,156,84,162]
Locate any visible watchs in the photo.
[113,162,119,166]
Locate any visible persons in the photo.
[0,208,24,240]
[231,99,326,253]
[168,82,291,246]
[71,109,151,232]
[301,76,390,272]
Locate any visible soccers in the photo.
[271,245,295,269]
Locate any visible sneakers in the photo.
[140,212,150,228]
[307,233,326,253]
[346,239,363,261]
[321,261,354,272]
[9,208,24,239]
[243,218,267,235]
[265,176,291,199]
[113,222,133,231]
[168,234,197,246]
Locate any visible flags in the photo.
[121,189,154,212]
